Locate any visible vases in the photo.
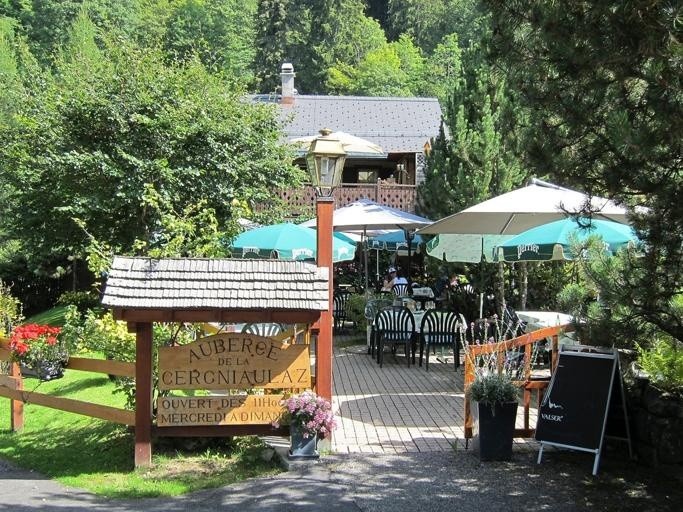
[472,400,518,461]
[289,425,318,458]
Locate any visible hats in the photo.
[388,267,396,274]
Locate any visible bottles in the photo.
[556,314,560,326]
[403,301,406,306]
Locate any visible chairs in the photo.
[333,283,465,371]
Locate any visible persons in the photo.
[386,174,395,183]
[384,270,409,297]
[388,267,396,278]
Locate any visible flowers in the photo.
[272,391,338,441]
[457,303,541,408]
[9,324,67,379]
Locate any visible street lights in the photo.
[305,127,346,456]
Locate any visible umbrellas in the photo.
[410,177,658,236]
[425,233,518,318]
[339,229,423,275]
[223,220,357,265]
[368,230,423,251]
[492,215,645,282]
[297,195,439,289]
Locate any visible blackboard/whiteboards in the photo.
[535,350,617,454]
[606,352,631,440]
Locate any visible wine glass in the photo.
[416,301,435,311]
